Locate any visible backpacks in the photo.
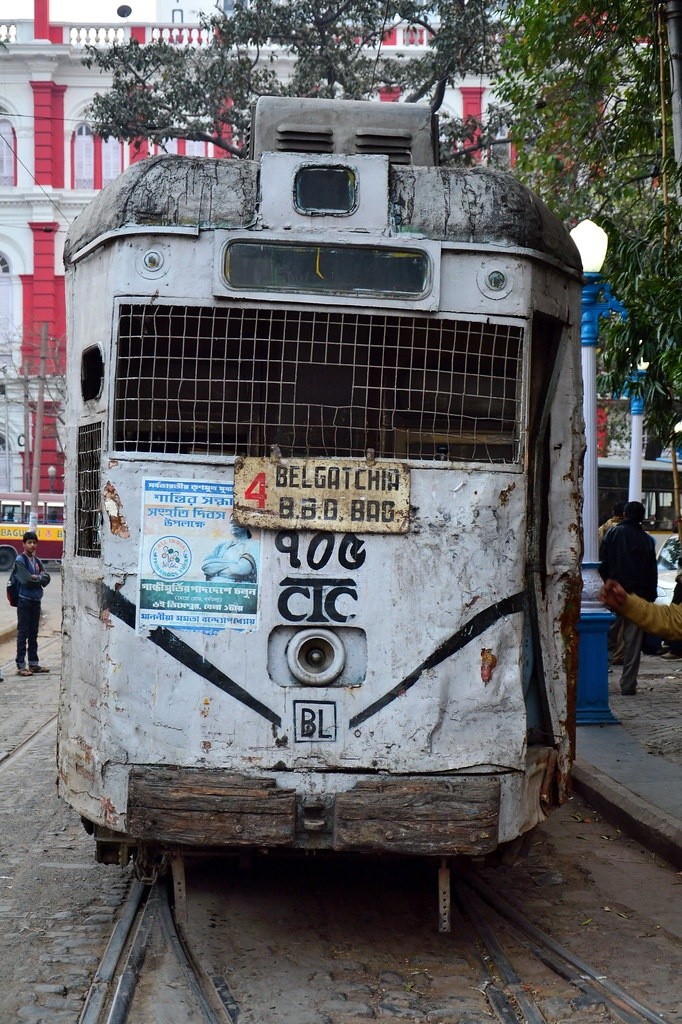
[6,554,38,607]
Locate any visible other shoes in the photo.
[621,689,637,696]
[660,652,682,662]
[29,665,50,673]
[17,668,33,676]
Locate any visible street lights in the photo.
[569,218,626,728]
[624,339,650,503]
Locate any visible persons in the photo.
[12,532,51,675]
[598,501,682,695]
[597,579,682,640]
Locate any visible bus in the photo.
[596,454,682,609]
[51,92,589,938]
[0,489,67,572]
